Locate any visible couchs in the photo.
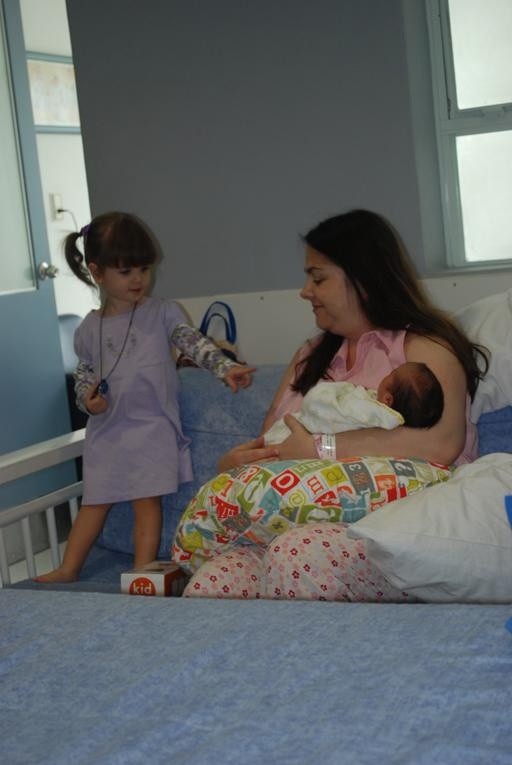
[0,272,511,601]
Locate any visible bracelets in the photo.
[314,435,337,461]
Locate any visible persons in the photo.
[183,207,479,599]
[32,212,256,583]
[259,362,439,446]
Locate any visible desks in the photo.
[0,588,511,764]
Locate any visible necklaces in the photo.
[96,301,137,394]
